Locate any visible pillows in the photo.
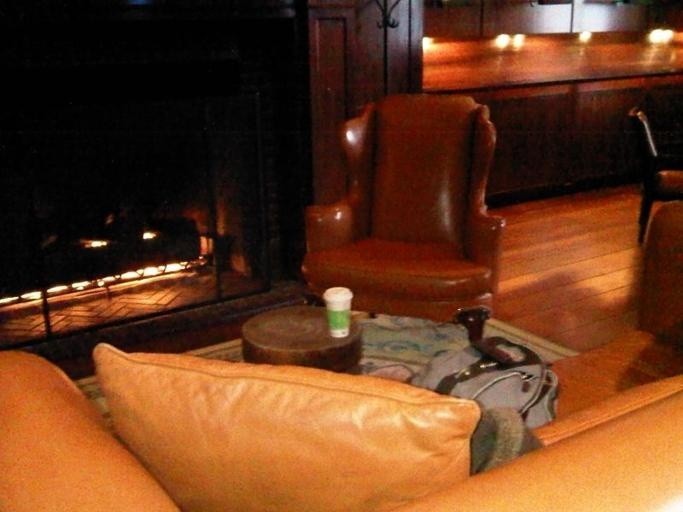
[91,338,480,512]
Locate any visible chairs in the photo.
[302,90,506,320]
[625,102,682,243]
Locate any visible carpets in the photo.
[71,300,580,438]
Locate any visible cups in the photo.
[321,286,354,339]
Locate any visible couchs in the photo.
[0,197,683,510]
